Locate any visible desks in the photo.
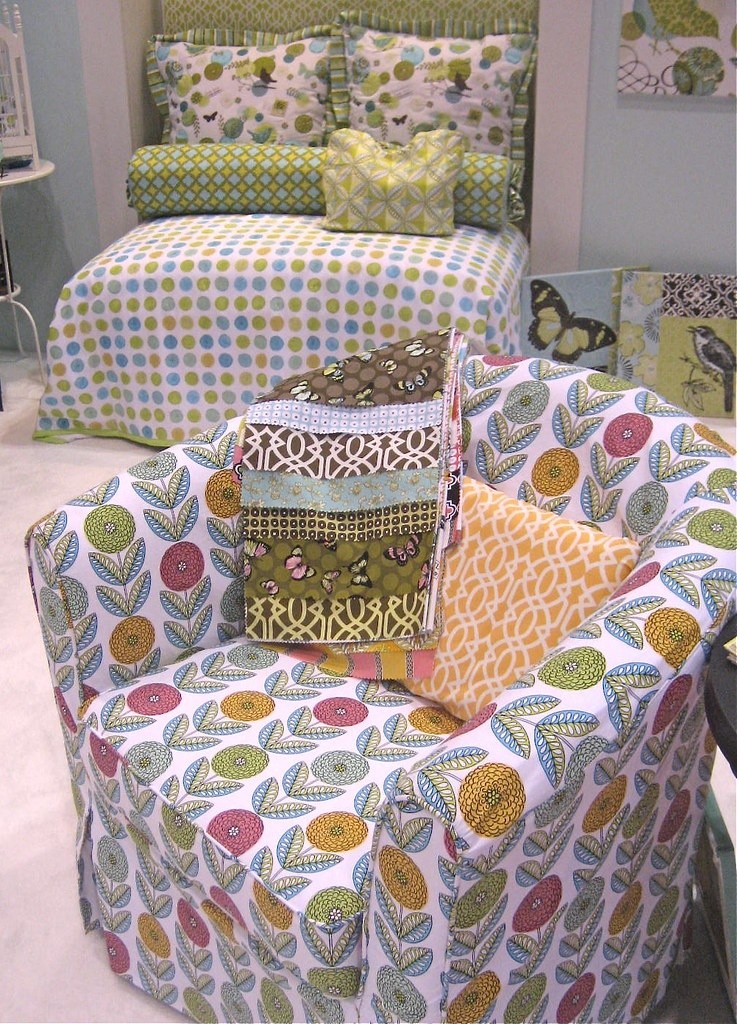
[0,159,55,386]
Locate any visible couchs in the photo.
[26,354,737,1024]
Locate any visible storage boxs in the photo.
[617,270,737,419]
[521,265,650,375]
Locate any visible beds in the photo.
[31,0,536,456]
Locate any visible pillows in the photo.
[321,128,464,236]
[332,10,538,222]
[125,144,525,232]
[392,476,644,722]
[149,25,338,147]
